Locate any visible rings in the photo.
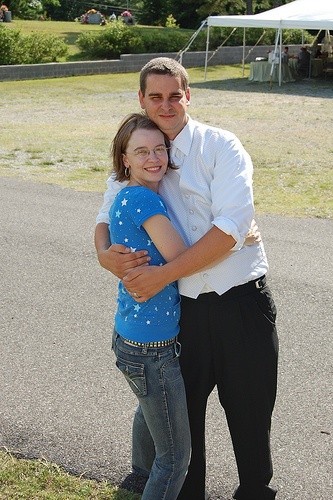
[134,292,140,299]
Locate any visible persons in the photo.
[93,57,279,500]
[281,47,289,83]
[113,113,263,500]
[80,10,133,26]
[296,45,329,77]
[0,2,8,21]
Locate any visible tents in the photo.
[207,0,333,86]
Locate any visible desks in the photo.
[250,60,299,83]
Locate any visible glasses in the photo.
[124,147,172,158]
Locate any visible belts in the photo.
[122,338,178,348]
[177,276,264,306]
